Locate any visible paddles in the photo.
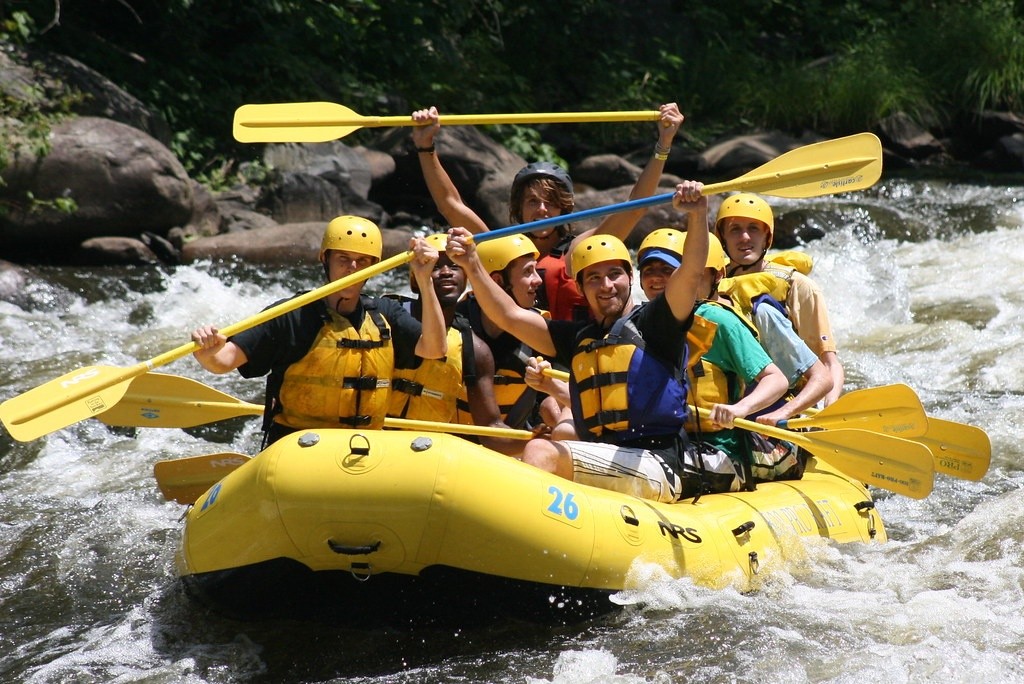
[93,369,534,442]
[462,131,884,246]
[153,452,253,507]
[542,367,937,501]
[779,383,929,441]
[232,99,667,144]
[1,248,416,442]
[803,403,991,484]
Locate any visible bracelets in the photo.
[414,141,435,156]
[653,142,671,161]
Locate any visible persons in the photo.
[411,102,684,372]
[381,192,845,501]
[445,181,709,505]
[193,215,448,452]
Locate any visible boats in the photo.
[175,428,888,627]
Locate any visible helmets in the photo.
[637,228,685,268]
[320,215,383,271]
[682,230,731,271]
[511,163,574,196]
[715,193,773,248]
[475,233,540,276]
[571,235,632,281]
[409,234,468,293]
